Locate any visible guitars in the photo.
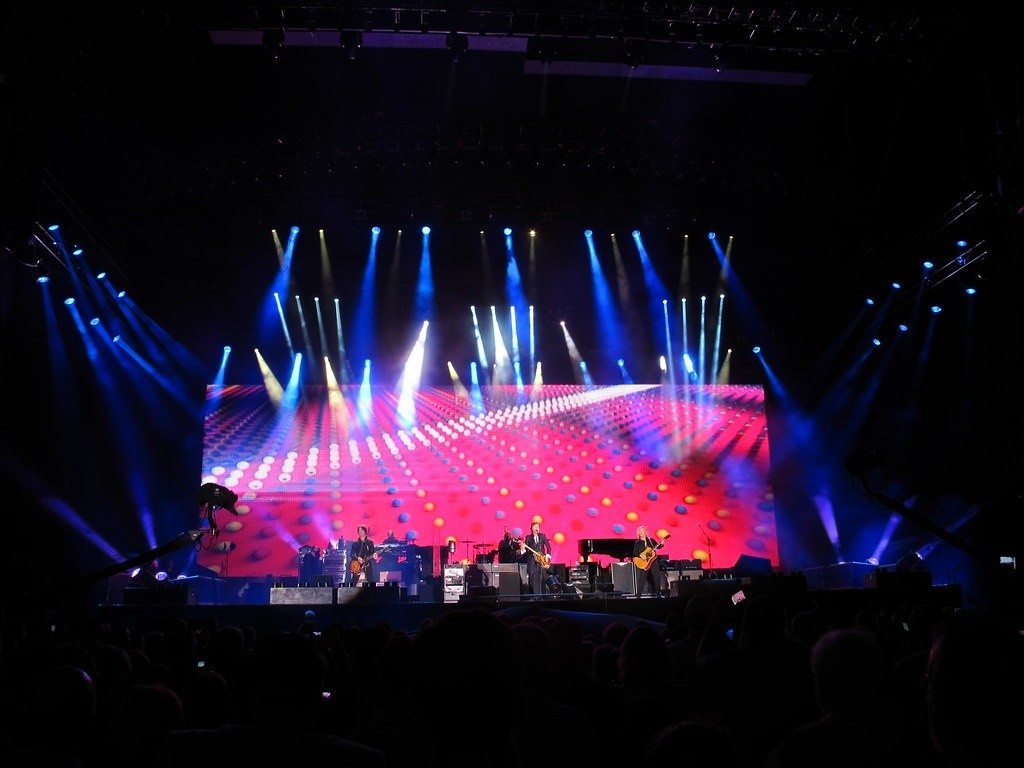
[516,536,551,570]
[349,544,392,575]
[632,534,673,571]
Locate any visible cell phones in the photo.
[731,590,746,605]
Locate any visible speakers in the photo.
[499,572,520,600]
[337,585,400,603]
[670,578,744,602]
[863,570,931,589]
[470,586,495,596]
[123,587,196,604]
[270,587,336,603]
[592,583,614,593]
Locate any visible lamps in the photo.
[155,571,172,592]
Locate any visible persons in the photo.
[632,526,664,598]
[383,530,400,544]
[498,530,521,563]
[0,583,1024,768]
[519,522,552,595]
[350,525,378,587]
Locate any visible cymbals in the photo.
[475,543,493,548]
[461,540,477,544]
